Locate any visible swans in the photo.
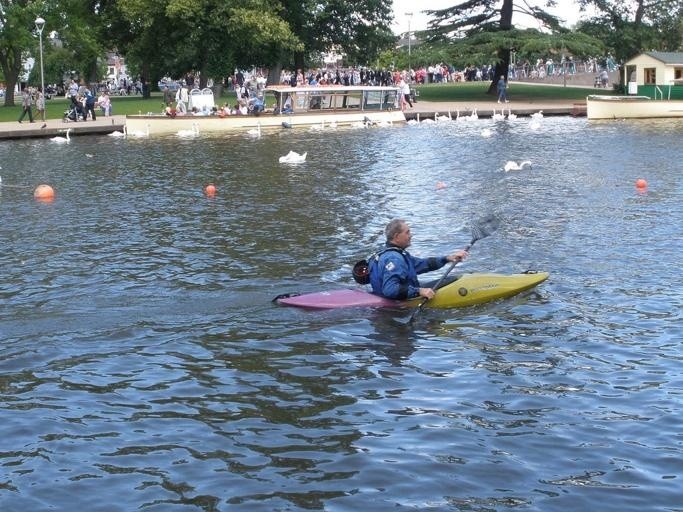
[131,123,150,140]
[407,108,545,130]
[504,160,532,172]
[174,121,201,138]
[278,150,307,164]
[308,119,393,132]
[107,125,126,137]
[247,123,260,135]
[49,128,71,143]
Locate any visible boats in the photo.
[586,77,683,118]
[276,270,551,310]
[125,85,406,135]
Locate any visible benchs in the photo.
[94,102,112,116]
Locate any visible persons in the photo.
[0,47,626,123]
[367,219,470,300]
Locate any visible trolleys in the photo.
[594,74,602,88]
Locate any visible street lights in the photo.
[404,12,413,71]
[618,55,627,96]
[561,55,567,88]
[509,45,521,71]
[34,16,45,93]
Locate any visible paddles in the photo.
[408,213,500,320]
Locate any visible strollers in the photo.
[64,94,83,123]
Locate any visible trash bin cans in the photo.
[188,88,214,111]
